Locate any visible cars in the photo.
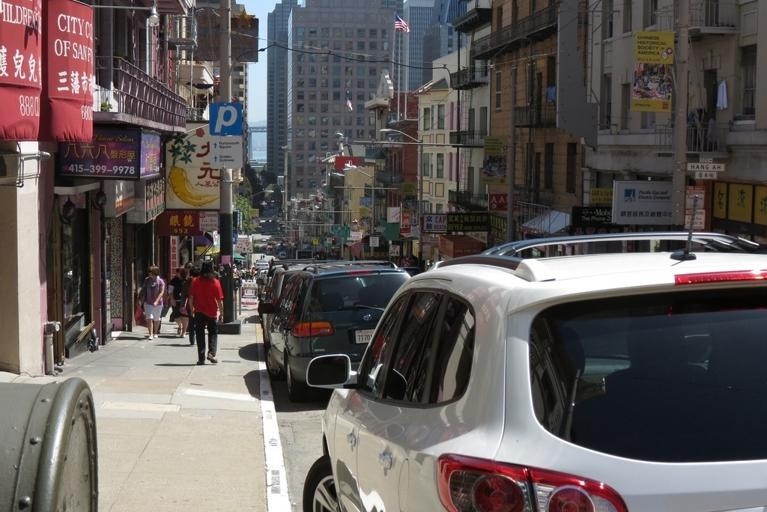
[304,230,767,512]
[248,252,413,403]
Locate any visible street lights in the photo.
[377,127,424,213]
[344,164,375,256]
[243,189,275,254]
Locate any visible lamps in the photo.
[90,4,160,27]
[59,195,75,226]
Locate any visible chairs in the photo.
[323,292,344,311]
[359,286,385,305]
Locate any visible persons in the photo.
[136,264,164,340]
[166,247,255,364]
